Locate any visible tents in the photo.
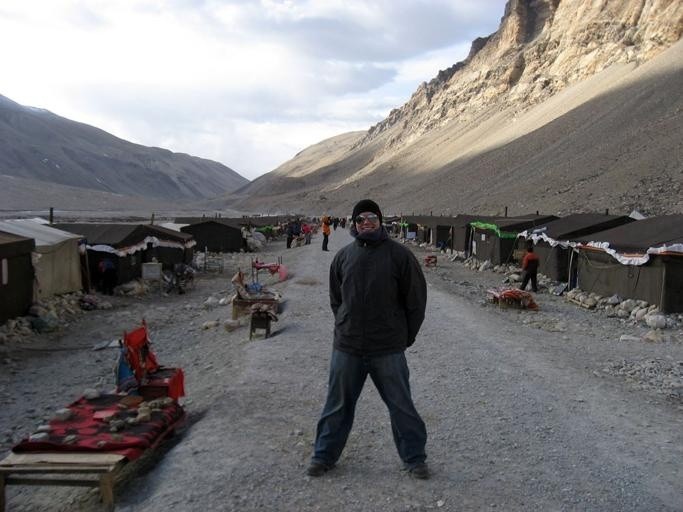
[0,217,299,326]
[381,214,560,266]
[516,211,637,283]
[564,211,682,315]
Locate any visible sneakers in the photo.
[306,459,335,476]
[409,464,428,478]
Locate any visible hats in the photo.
[352,199,382,224]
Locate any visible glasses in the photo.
[353,213,379,225]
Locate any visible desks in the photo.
[0,395,176,512]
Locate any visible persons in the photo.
[519,245,540,292]
[286,214,346,251]
[306,199,431,480]
